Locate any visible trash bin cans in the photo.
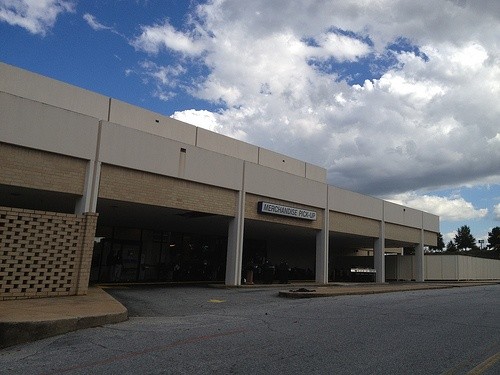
[277,263,291,284]
[262,263,276,285]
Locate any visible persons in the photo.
[113,251,123,282]
[107,249,116,281]
